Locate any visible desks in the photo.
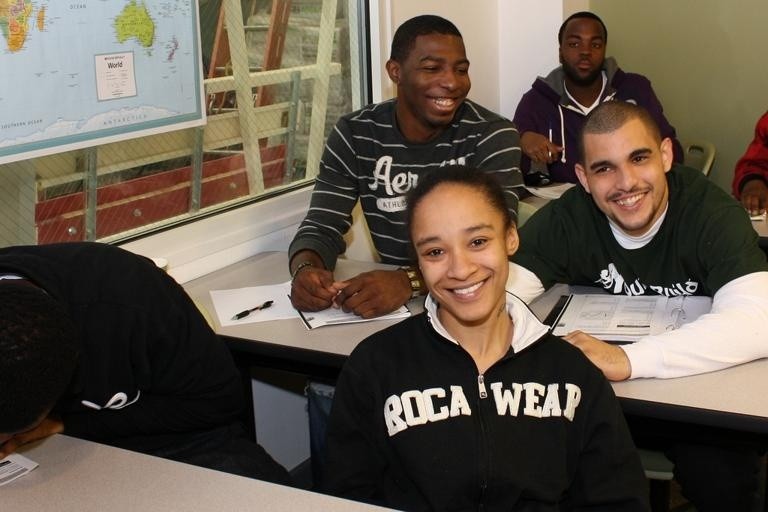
[0,424,415,512]
[517,179,767,236]
[175,249,767,423]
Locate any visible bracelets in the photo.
[397,266,421,300]
[290,260,317,285]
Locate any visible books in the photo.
[548,286,706,344]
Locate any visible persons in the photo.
[504,101,768,383]
[0,242,291,486]
[732,110,768,216]
[318,164,651,512]
[512,11,685,184]
[288,14,527,322]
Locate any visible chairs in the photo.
[674,136,718,177]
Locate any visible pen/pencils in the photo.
[230,300,274,321]
[548,127,552,158]
